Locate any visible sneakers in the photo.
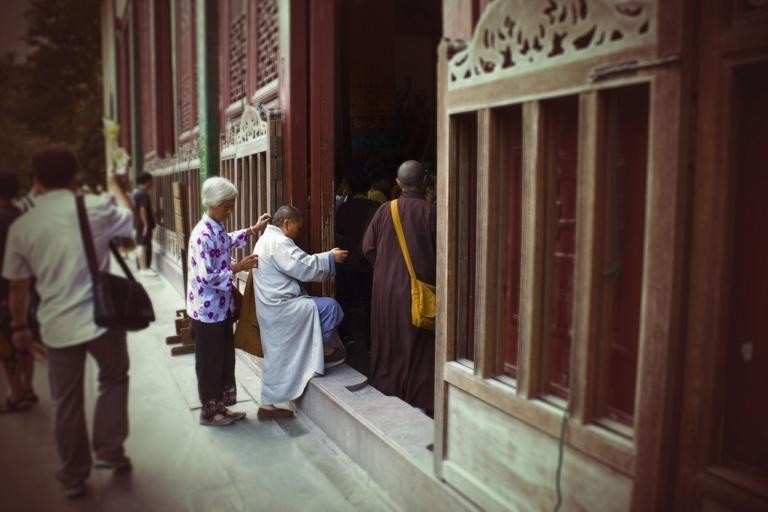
[220,408,247,421]
[199,413,235,427]
[25,389,39,403]
[1,396,33,411]
[256,406,294,420]
[94,446,132,475]
[58,477,85,498]
[321,348,347,364]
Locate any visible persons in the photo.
[0,172,156,413]
[252,205,350,422]
[185,176,271,427]
[333,160,436,420]
[3,144,131,500]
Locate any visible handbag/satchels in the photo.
[230,268,269,360]
[85,268,157,333]
[228,281,243,321]
[406,275,436,333]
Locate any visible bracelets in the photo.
[250,225,257,235]
